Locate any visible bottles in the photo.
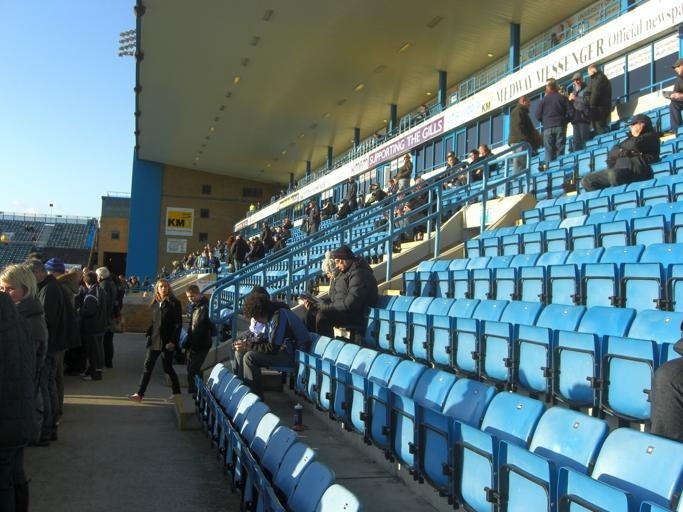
[293,402,304,431]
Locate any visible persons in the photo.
[551,23,565,45]
[581,114,660,190]
[128,279,183,402]
[231,245,379,399]
[670,58,683,130]
[374,133,383,140]
[159,217,293,279]
[128,277,141,289]
[143,276,151,286]
[365,144,495,250]
[305,177,358,238]
[417,104,430,117]
[1,252,125,511]
[184,285,215,395]
[508,64,611,176]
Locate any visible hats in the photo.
[672,59,683,68]
[44,258,65,272]
[626,114,649,126]
[333,245,355,259]
[571,73,583,81]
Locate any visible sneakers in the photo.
[165,394,184,402]
[664,130,678,136]
[28,414,64,447]
[258,393,264,401]
[128,394,142,402]
[64,368,104,380]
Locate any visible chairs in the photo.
[0,220,89,268]
